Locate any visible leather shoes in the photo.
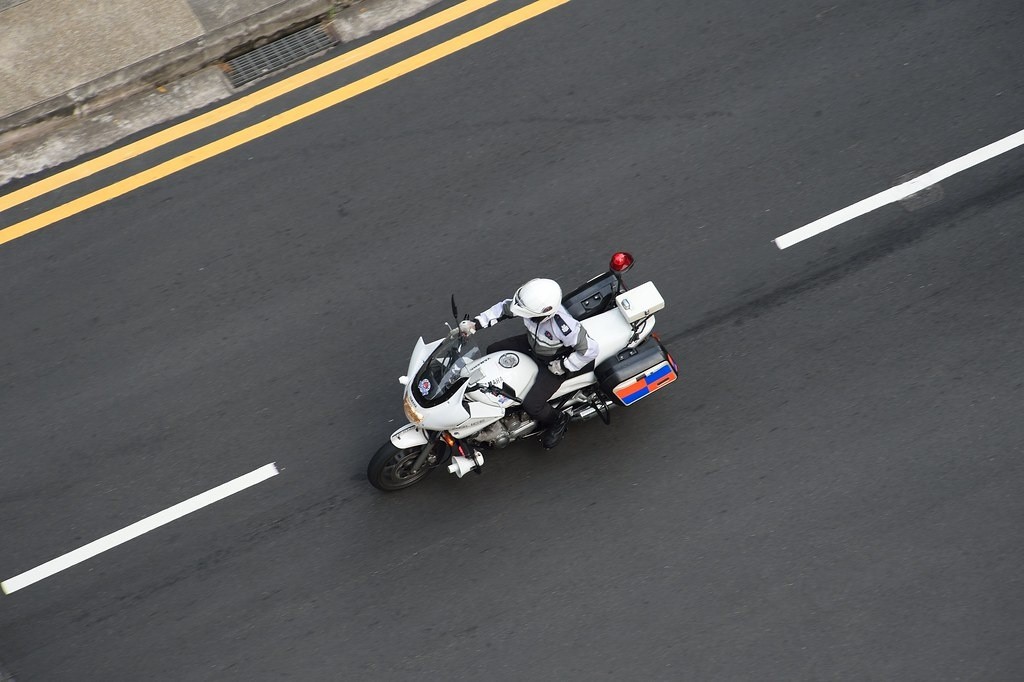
[543,411,571,449]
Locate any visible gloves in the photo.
[450,320,476,337]
[548,361,565,376]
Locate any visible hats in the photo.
[510,277,563,324]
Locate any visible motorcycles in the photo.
[366,248,679,495]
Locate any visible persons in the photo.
[460,278,600,449]
